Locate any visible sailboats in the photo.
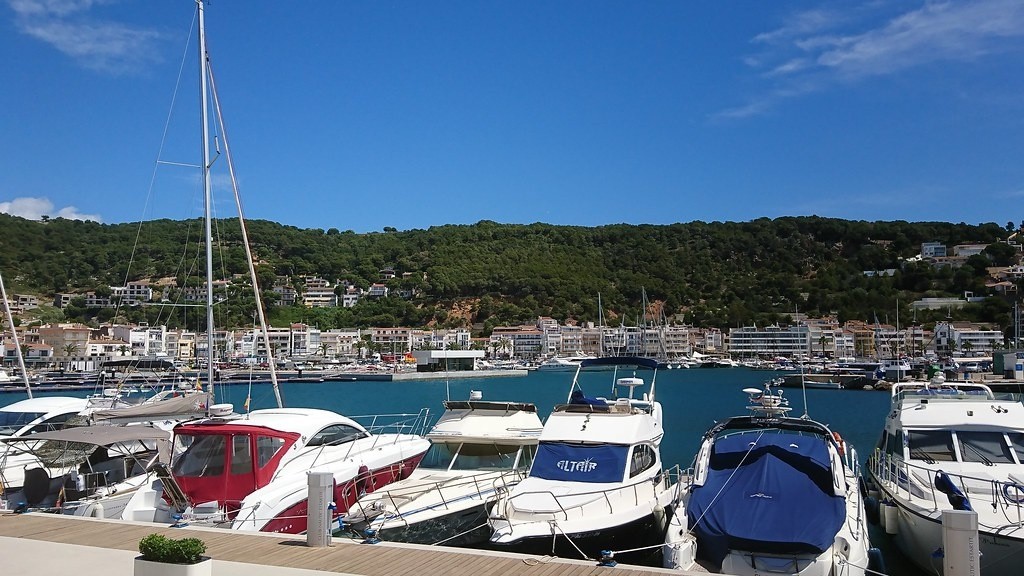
[0,0,284,576]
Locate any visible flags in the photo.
[195,375,203,390]
[243,394,249,411]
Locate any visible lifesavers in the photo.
[832,431,844,457]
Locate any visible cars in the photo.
[981,363,992,372]
[177,353,417,371]
[964,361,983,372]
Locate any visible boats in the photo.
[0,425,171,518]
[866,370,1024,576]
[483,356,681,546]
[120,402,433,536]
[539,286,732,368]
[661,304,886,576]
[337,346,545,546]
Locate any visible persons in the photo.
[393,363,399,374]
